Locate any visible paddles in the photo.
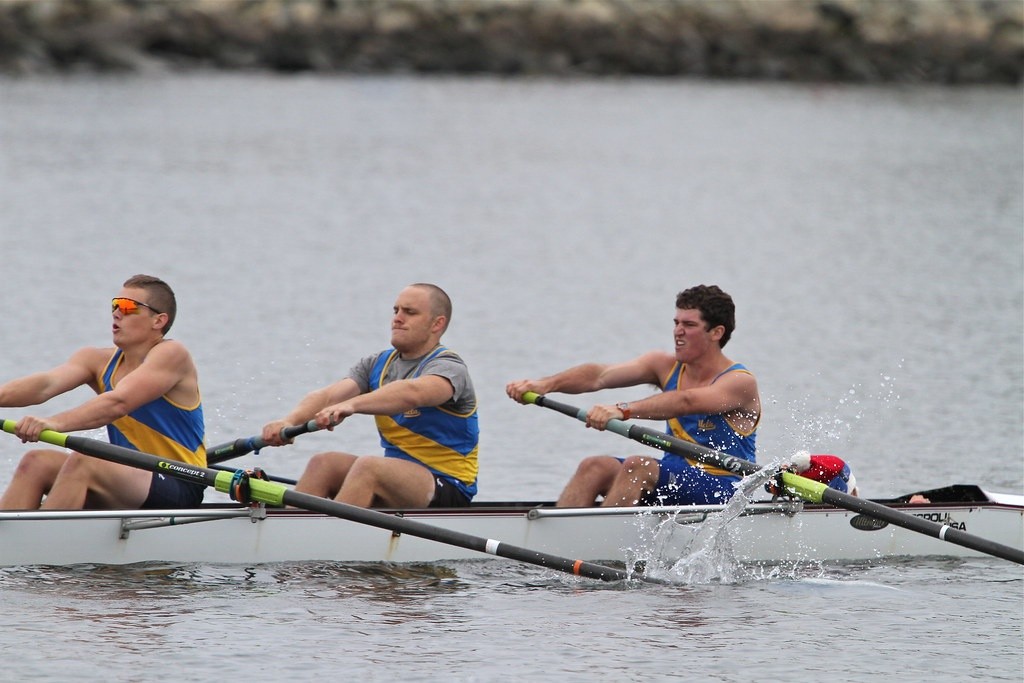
[205,414,346,467]
[0,419,695,588]
[520,392,1024,567]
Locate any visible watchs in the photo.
[616,400,630,421]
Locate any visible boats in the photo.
[0,485,1024,566]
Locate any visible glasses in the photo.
[111,298,161,314]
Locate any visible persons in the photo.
[263,285,480,510]
[791,456,930,505]
[506,286,763,509]
[1,275,208,510]
